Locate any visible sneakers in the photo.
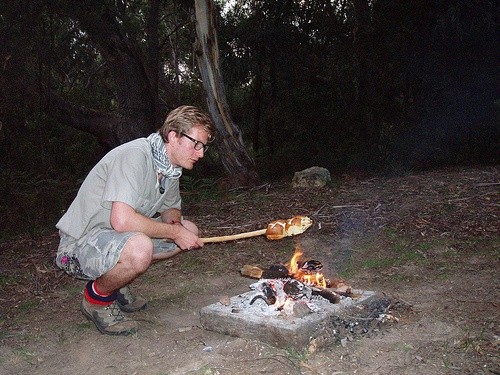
[80,292,139,336]
[115,284,148,313]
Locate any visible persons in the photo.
[54,105,219,338]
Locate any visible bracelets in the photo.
[170,219,185,227]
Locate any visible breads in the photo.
[267,216,312,240]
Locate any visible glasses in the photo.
[182,133,209,153]
